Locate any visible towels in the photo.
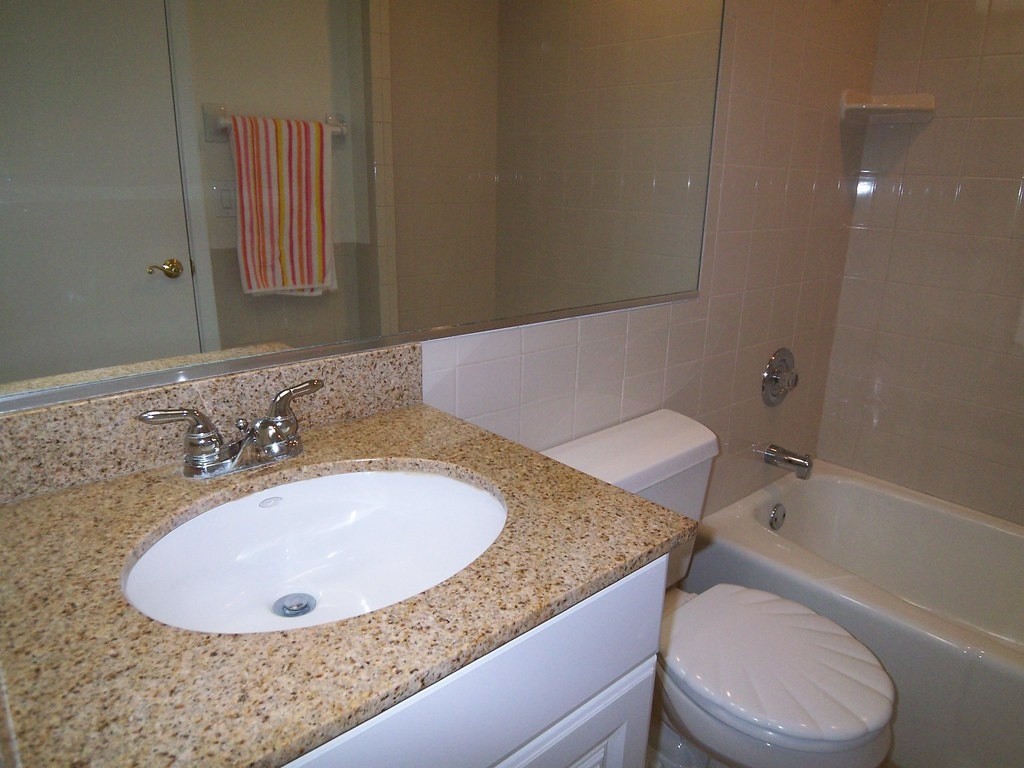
[228,115,338,296]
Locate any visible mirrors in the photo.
[0,0,724,416]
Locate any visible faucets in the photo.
[138,379,325,479]
[764,443,812,480]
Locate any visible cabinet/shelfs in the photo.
[494,655,658,768]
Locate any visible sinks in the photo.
[115,454,509,632]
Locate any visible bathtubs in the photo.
[679,456,1023,768]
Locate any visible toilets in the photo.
[537,409,900,768]
